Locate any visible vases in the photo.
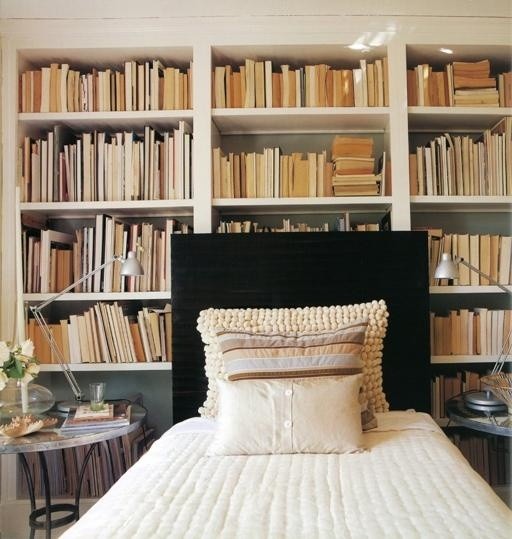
[0,380,55,418]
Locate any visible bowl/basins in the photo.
[479,374,512,411]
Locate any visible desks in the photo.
[445,387,512,438]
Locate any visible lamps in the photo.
[30,249,145,413]
[433,251,512,413]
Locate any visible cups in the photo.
[88,382,106,412]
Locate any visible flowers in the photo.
[0,337,41,390]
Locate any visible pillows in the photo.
[215,317,378,430]
[204,372,371,456]
[196,299,390,419]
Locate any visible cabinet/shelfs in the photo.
[0,15,512,539]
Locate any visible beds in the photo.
[54,229,512,539]
